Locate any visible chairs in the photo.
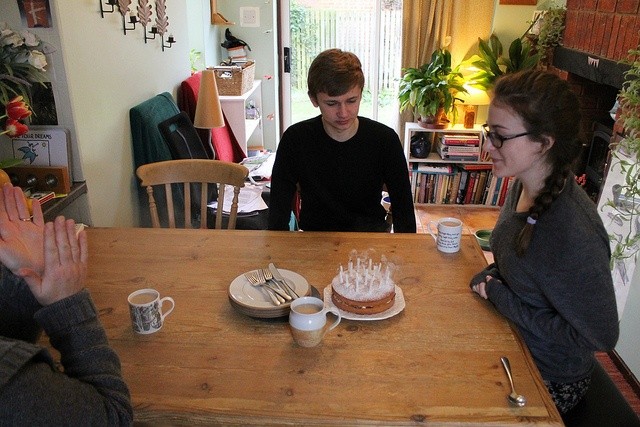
[135,159,248,229]
[157,111,271,229]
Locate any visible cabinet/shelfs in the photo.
[404,122,521,209]
[206,80,263,162]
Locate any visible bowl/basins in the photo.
[474,229,493,251]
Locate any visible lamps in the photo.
[454,72,491,129]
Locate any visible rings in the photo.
[21,218,30,221]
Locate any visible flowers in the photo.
[0,22,52,126]
[0,94,33,170]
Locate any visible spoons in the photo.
[500,355,527,406]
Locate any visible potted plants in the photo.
[398,47,468,129]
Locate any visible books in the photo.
[434,133,480,161]
[409,163,515,206]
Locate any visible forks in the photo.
[249,271,281,305]
[243,271,292,300]
[256,268,286,303]
[262,265,286,293]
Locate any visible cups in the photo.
[127,288,175,334]
[288,296,342,348]
[427,217,462,253]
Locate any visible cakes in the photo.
[329,269,396,315]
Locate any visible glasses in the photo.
[482,122,557,149]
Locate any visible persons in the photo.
[268,48,416,233]
[0,169,133,427]
[470,69,619,415]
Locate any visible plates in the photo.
[323,282,405,320]
[228,267,312,318]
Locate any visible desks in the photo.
[3,180,90,224]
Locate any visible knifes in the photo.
[269,262,300,299]
